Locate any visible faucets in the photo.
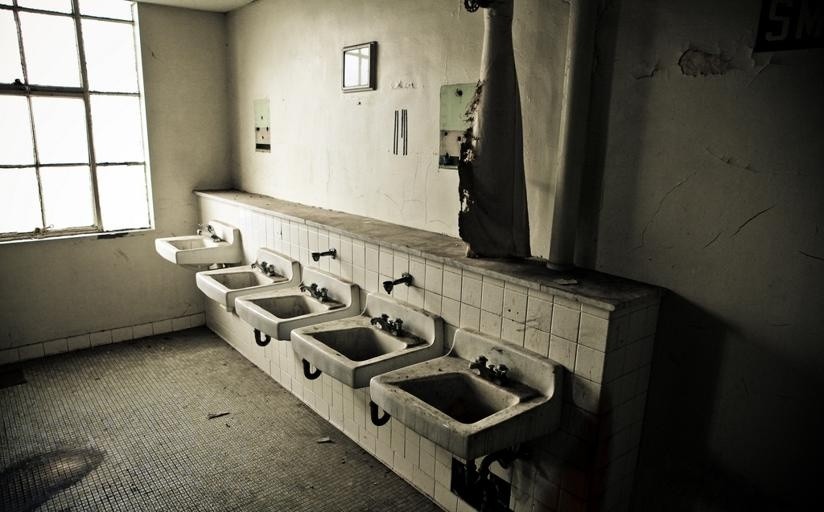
[470,362,496,379]
[301,286,321,298]
[197,229,213,237]
[370,318,393,332]
[251,263,266,273]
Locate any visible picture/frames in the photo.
[340,41,378,95]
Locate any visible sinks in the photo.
[234,286,350,341]
[371,354,551,461]
[155,234,232,265]
[195,264,290,313]
[291,315,431,389]
